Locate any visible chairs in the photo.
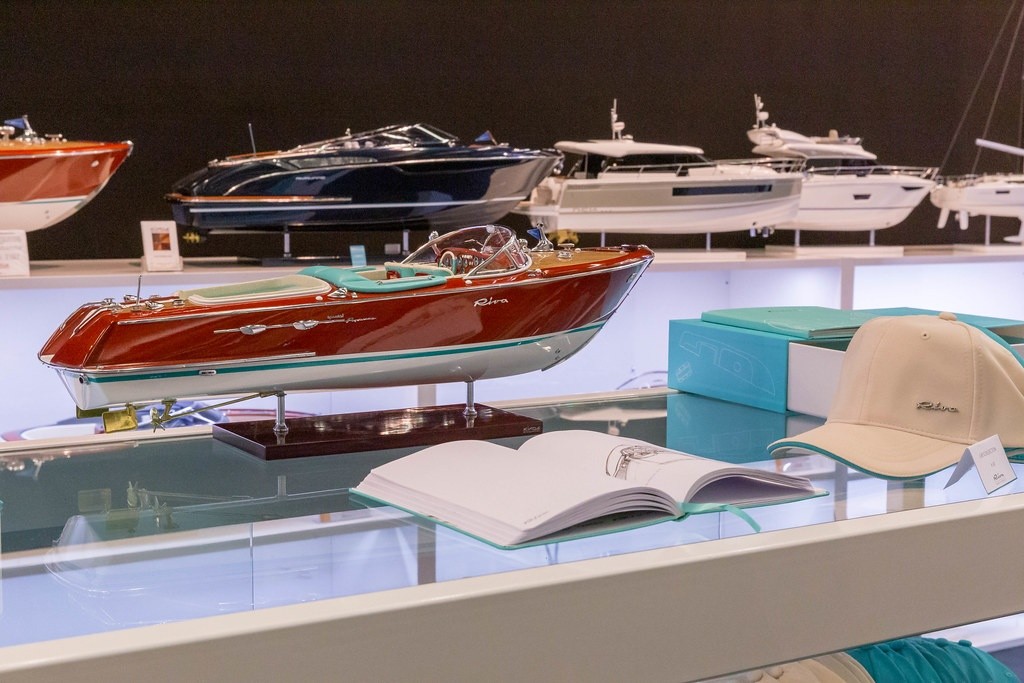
[414,265,454,276]
[384,262,413,277]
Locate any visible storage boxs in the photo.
[668,307,1024,423]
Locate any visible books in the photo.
[349,429,830,550]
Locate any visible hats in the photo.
[767,311,1024,482]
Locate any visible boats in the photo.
[0,115,131,230]
[931,136,1024,244]
[712,94,937,230]
[510,97,804,234]
[164,122,563,233]
[2,403,321,449]
[35,219,653,411]
